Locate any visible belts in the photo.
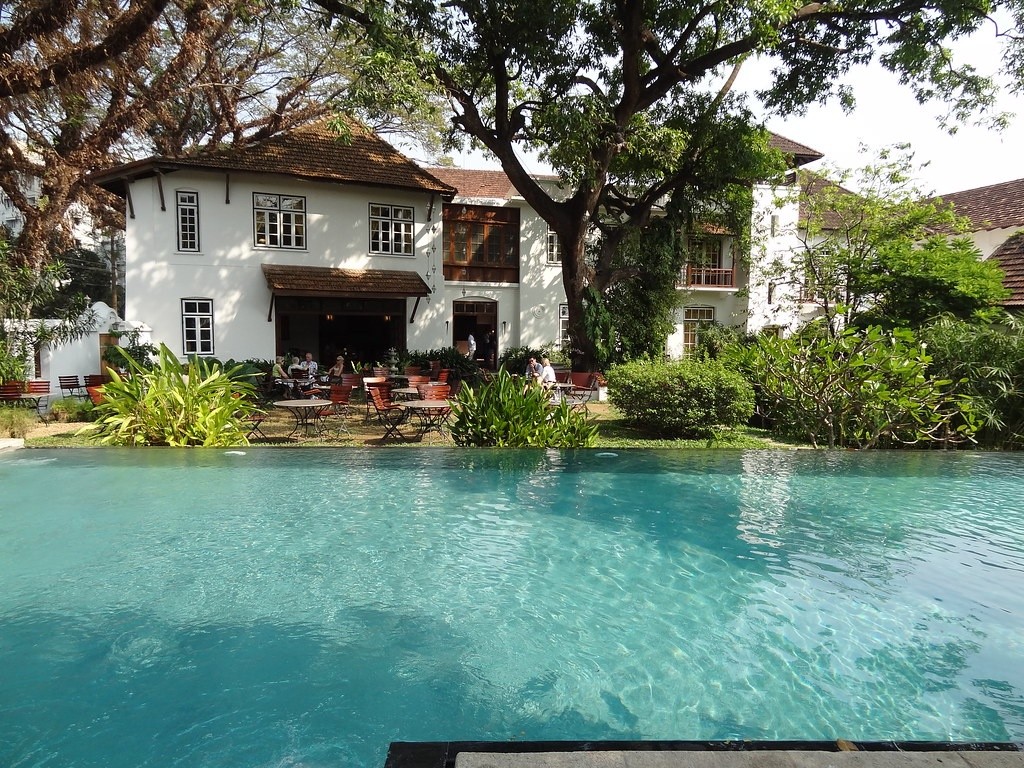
[545,380,556,384]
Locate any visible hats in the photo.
[337,356,344,360]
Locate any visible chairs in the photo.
[365,382,392,421]
[363,376,386,420]
[231,393,269,442]
[416,384,453,433]
[253,360,463,409]
[370,387,412,443]
[557,370,572,398]
[571,373,600,414]
[316,386,352,439]
[0,374,128,428]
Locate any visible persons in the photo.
[328,356,344,377]
[300,353,318,400]
[523,357,556,395]
[288,357,307,378]
[272,356,293,389]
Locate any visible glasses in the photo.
[529,360,533,362]
[336,359,339,361]
[282,360,284,362]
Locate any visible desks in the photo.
[542,382,574,405]
[279,379,308,400]
[388,373,409,387]
[272,400,333,442]
[429,382,448,386]
[391,388,419,419]
[401,400,458,441]
[513,376,533,386]
